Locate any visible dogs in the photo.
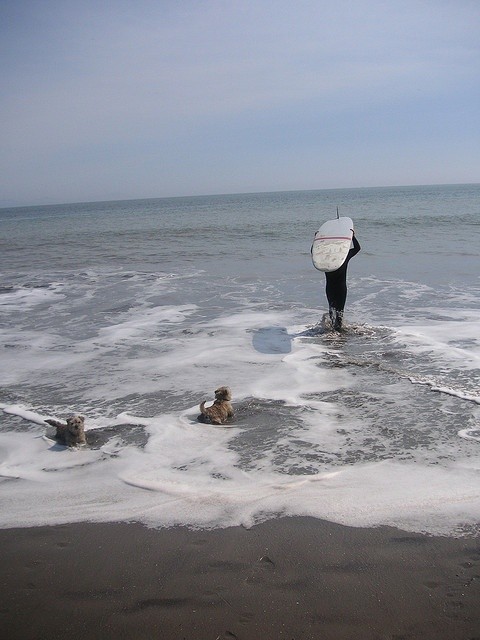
[44,416,88,447]
[199,385,236,425]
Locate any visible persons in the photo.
[311,229,360,332]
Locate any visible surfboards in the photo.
[311,206,354,272]
